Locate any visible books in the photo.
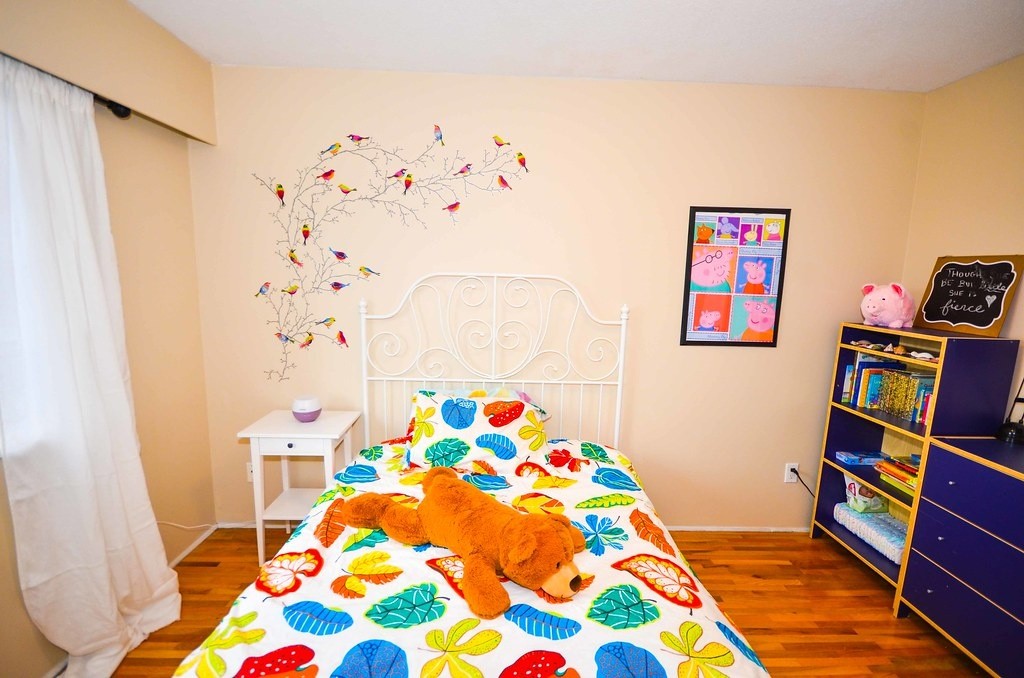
[849,350,936,427]
[873,452,922,497]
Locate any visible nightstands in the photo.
[236,408,361,567]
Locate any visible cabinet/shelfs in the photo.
[808,321,1021,610]
[892,435,1023,678]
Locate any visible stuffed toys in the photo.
[861,282,916,328]
[343,467,585,620]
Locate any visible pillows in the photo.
[403,389,552,476]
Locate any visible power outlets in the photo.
[246,462,254,481]
[784,462,799,482]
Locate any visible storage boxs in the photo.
[836,452,891,465]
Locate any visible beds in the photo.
[172,271,771,677]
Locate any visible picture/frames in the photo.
[679,205,791,348]
